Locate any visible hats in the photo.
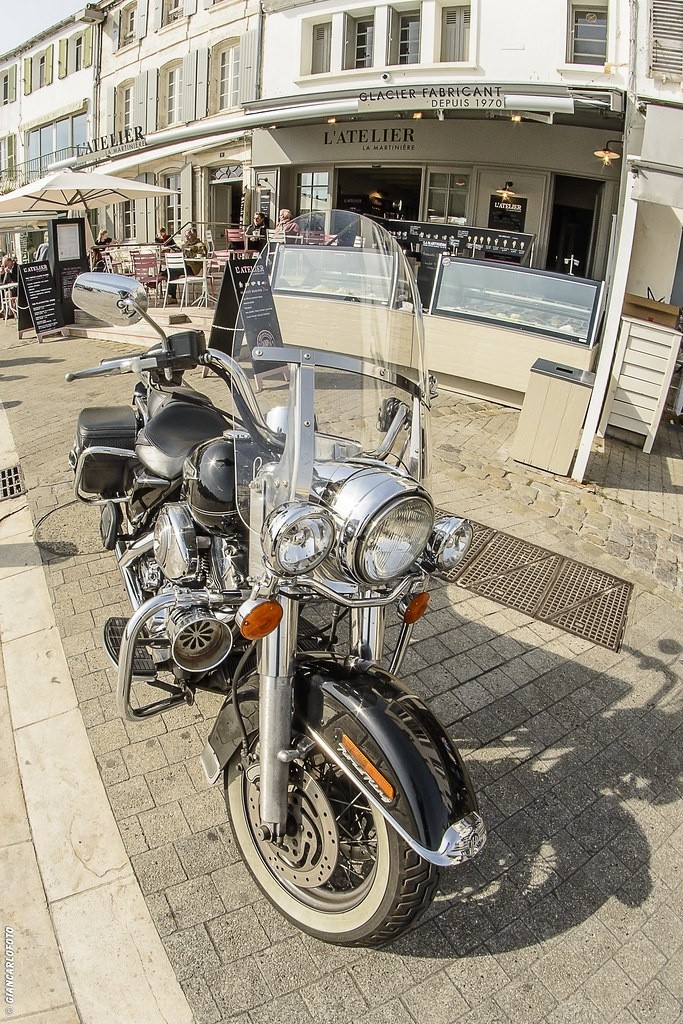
[369,191,382,199]
[1,258,11,266]
[159,228,166,232]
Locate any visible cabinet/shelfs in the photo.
[595,315,683,454]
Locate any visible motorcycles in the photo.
[64,208,487,949]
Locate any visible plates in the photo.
[194,257,202,258]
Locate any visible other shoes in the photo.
[161,297,177,304]
[3,314,14,320]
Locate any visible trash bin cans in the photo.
[509,356,597,477]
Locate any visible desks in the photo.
[0,282,20,326]
[184,258,218,309]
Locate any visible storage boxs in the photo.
[70,406,137,494]
[623,293,679,328]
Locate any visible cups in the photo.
[253,231,260,236]
[197,254,202,257]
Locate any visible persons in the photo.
[0,232,48,320]
[226,208,301,276]
[154,228,176,246]
[94,229,119,246]
[144,228,206,304]
[86,246,104,272]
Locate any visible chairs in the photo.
[101,227,324,313]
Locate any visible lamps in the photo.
[497,181,516,197]
[437,109,445,121]
[74,2,108,25]
[593,140,623,165]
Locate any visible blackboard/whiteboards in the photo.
[13,260,66,335]
[207,258,288,378]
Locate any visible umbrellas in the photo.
[0,166,183,228]
